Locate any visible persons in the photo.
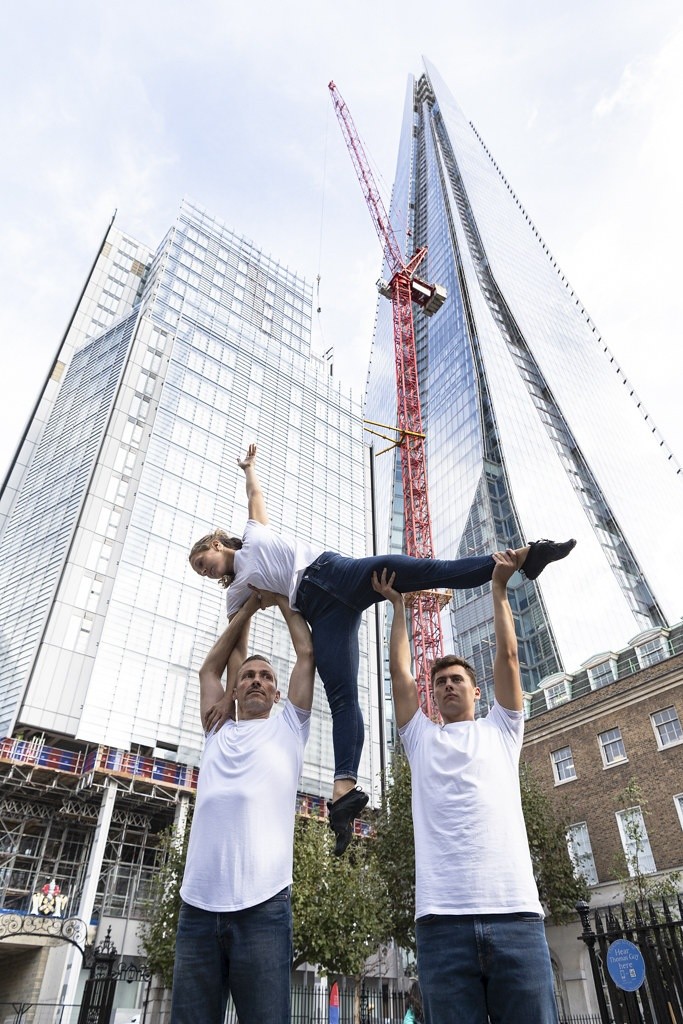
[188,444,579,859]
[171,584,317,1024]
[371,548,561,1024]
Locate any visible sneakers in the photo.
[517,538,577,581]
[326,786,369,856]
